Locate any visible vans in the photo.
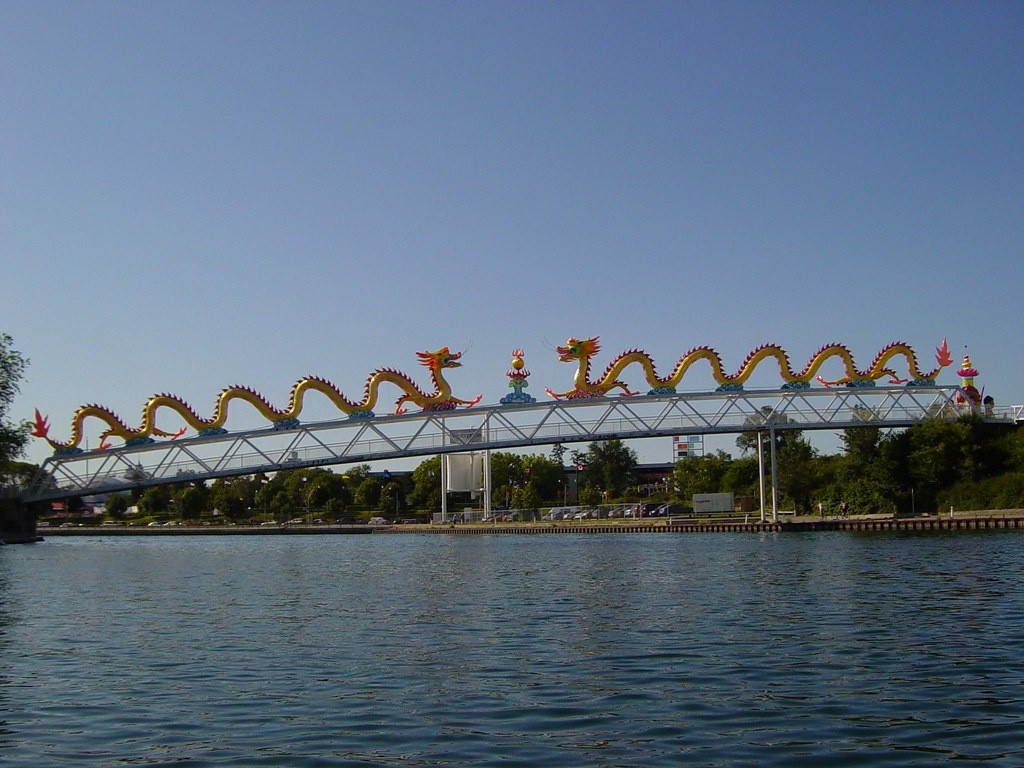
[541,506,580,521]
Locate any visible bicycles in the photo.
[836,510,851,520]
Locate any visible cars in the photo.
[148,520,236,526]
[563,502,694,519]
[482,513,513,522]
[261,519,280,525]
[287,517,307,524]
[333,517,390,525]
[312,518,328,524]
[35,522,96,528]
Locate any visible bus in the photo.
[102,521,127,526]
